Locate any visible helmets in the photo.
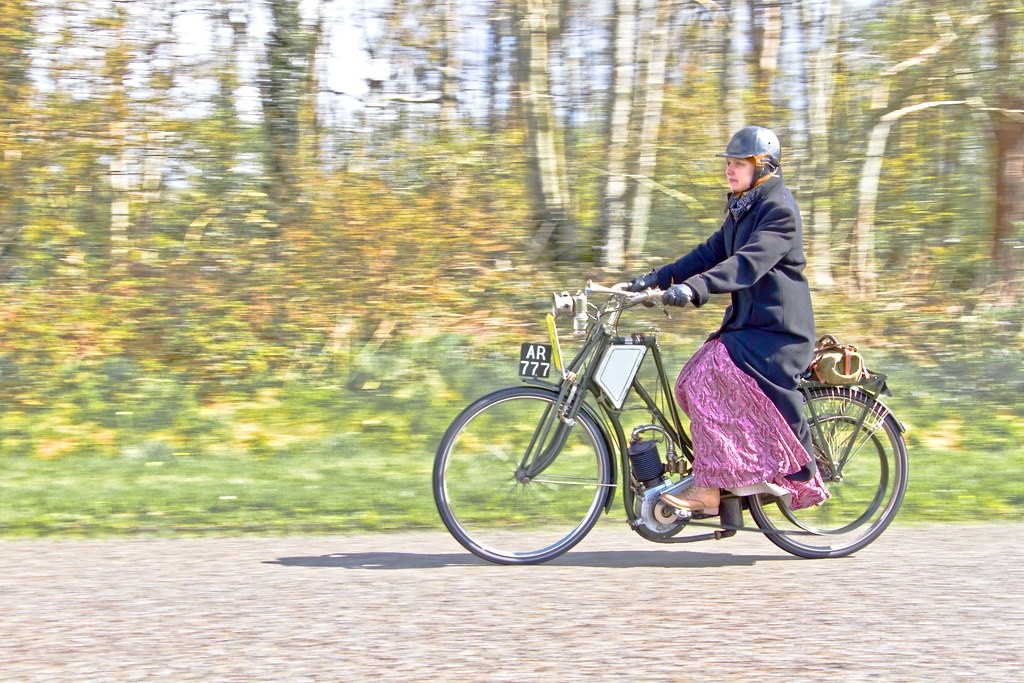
[715,126,781,174]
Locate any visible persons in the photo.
[628,126,831,515]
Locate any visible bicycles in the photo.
[430,281,908,567]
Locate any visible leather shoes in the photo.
[659,485,720,515]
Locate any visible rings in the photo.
[668,290,676,298]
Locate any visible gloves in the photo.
[629,271,658,292]
[662,283,696,307]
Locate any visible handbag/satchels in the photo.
[810,334,870,385]
